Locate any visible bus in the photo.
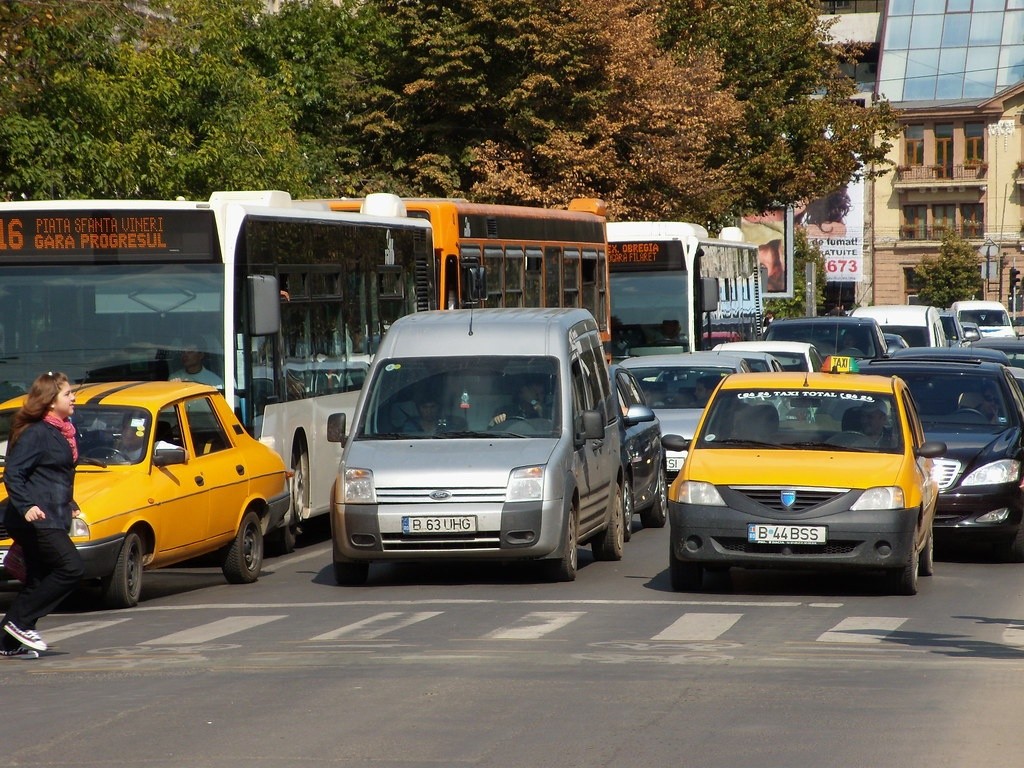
[296,198,612,368]
[0,190,438,552]
[606,222,764,363]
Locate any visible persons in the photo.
[976,386,1002,425]
[112,414,190,462]
[168,336,224,390]
[694,378,714,400]
[0,370,84,660]
[487,373,553,428]
[842,331,856,348]
[860,398,898,453]
[403,392,458,435]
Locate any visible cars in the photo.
[655,340,826,425]
[0,381,295,608]
[854,336,1024,549]
[619,354,751,488]
[606,364,667,543]
[937,312,983,348]
[667,356,947,596]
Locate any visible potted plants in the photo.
[901,223,915,232]
[963,157,982,170]
[897,163,912,171]
[930,164,945,170]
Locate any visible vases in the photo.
[966,225,982,229]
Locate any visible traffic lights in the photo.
[1010,267,1020,292]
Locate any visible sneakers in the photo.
[0,646,38,659]
[4,621,48,652]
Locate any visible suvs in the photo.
[762,317,888,363]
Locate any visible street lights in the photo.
[999,259,1009,302]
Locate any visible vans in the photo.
[949,301,1016,338]
[847,305,947,348]
[327,307,623,584]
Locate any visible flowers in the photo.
[964,219,983,225]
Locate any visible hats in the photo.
[853,399,887,415]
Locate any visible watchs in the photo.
[531,399,539,406]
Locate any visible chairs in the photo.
[732,404,780,442]
[167,332,222,374]
[843,406,862,432]
[156,421,174,444]
